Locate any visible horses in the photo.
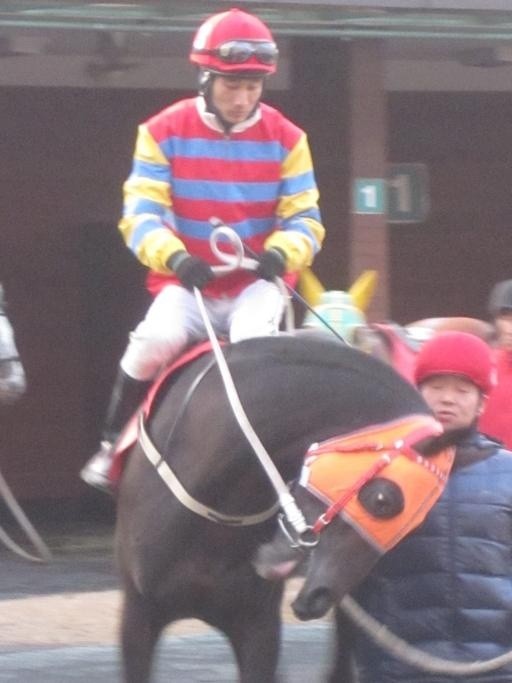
[111,334,500,683]
[298,264,504,397]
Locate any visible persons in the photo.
[339,331,512,683]
[81,8,328,493]
[482,279,512,452]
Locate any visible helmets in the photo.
[414,332,497,394]
[190,8,278,73]
[488,279,512,313]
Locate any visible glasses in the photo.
[191,39,279,64]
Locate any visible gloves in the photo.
[255,247,287,282]
[166,249,214,291]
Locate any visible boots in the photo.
[81,362,153,495]
